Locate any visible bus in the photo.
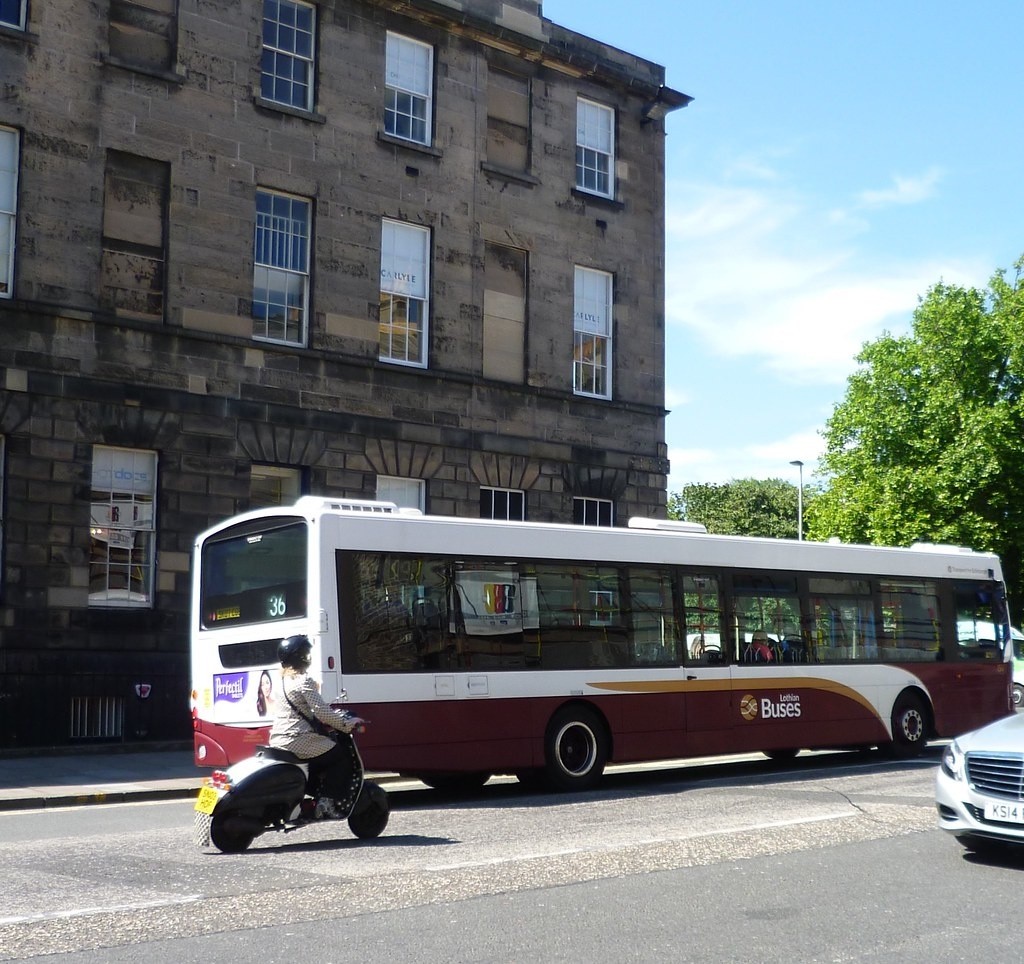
[189,495,1017,796]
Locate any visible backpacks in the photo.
[746,646,761,665]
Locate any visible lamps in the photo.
[90,528,96,534]
[97,529,102,534]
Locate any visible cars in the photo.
[936,712,1024,856]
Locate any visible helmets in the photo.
[279,634,309,662]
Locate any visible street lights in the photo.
[788,460,805,543]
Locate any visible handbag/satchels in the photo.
[314,720,331,739]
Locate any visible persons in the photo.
[257,669,275,717]
[744,630,774,666]
[268,635,366,822]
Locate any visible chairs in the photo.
[411,597,939,662]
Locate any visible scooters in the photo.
[194,686,390,852]
